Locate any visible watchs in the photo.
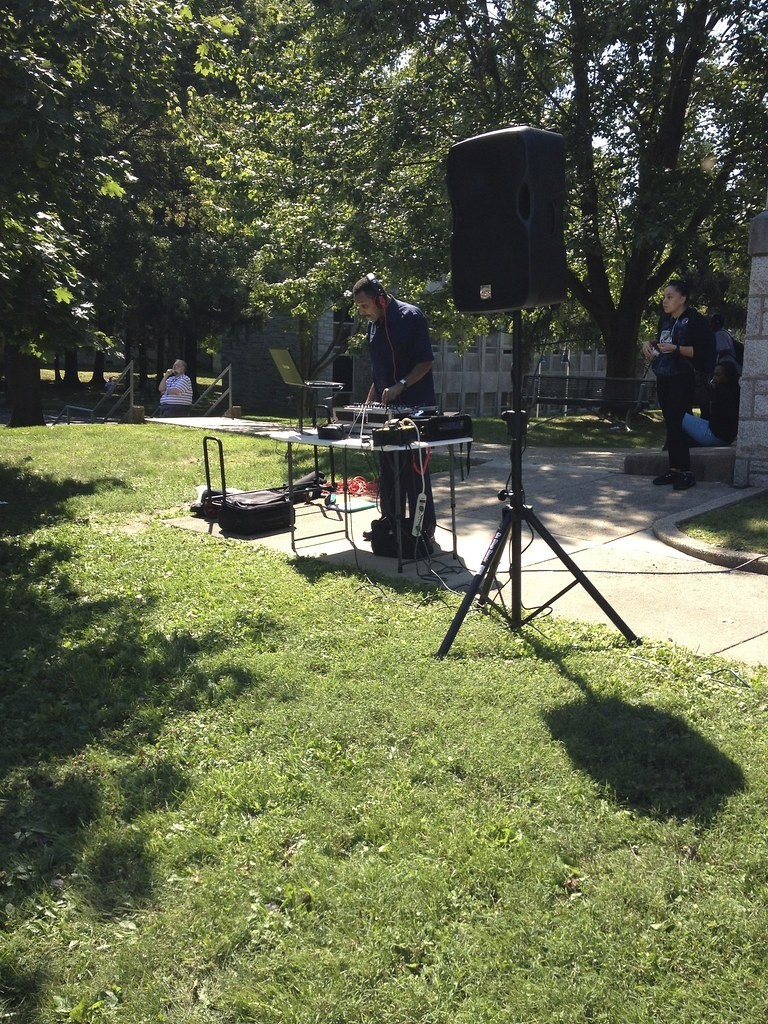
[399,379,407,391]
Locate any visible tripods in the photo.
[435,311,642,661]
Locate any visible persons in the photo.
[158,360,193,417]
[709,314,742,378]
[663,361,740,446]
[105,378,116,400]
[353,277,436,544]
[642,281,707,490]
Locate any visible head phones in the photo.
[366,272,387,310]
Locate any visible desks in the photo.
[269,427,473,574]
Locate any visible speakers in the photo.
[446,126,564,314]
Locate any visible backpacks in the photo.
[722,330,744,366]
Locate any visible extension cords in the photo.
[412,493,426,536]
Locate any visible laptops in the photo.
[269,347,344,387]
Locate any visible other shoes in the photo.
[673,472,696,489]
[653,468,676,485]
[363,529,372,538]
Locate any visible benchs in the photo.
[521,375,659,432]
